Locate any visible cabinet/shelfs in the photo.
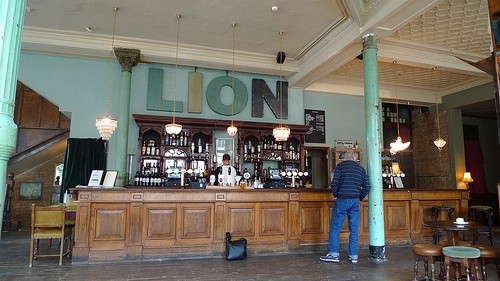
[133,113,309,186]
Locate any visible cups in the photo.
[456,218,463,223]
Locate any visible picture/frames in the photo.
[304,109,326,142]
[19,181,43,201]
[51,193,61,206]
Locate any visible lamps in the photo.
[165,14,182,135]
[390,60,411,155]
[96,7,118,141]
[390,162,403,187]
[432,67,446,151]
[276,52,286,64]
[462,172,473,188]
[272,32,291,141]
[227,21,238,135]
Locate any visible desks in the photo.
[38,205,78,258]
[424,220,481,244]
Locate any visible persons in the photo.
[319,147,371,262]
[213,154,241,186]
[54,175,61,185]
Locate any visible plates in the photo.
[453,222,469,224]
[235,136,311,189]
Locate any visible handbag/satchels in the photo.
[225,231,247,261]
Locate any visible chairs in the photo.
[28,203,71,265]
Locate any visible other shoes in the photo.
[348,256,357,263]
[319,253,340,262]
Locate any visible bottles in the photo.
[180,138,222,187]
[140,140,160,156]
[134,161,165,187]
[161,131,188,147]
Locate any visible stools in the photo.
[412,205,500,281]
